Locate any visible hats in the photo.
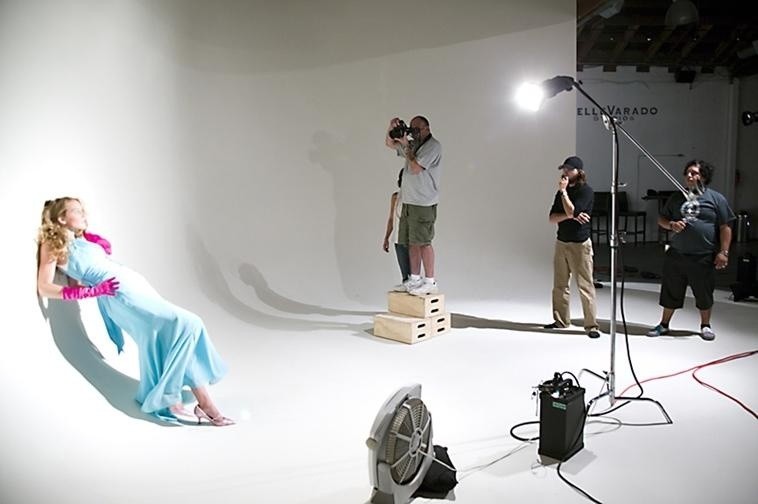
[559,156,583,170]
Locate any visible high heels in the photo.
[194,404,235,426]
[170,403,193,416]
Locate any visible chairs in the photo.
[615,191,647,241]
[588,192,611,246]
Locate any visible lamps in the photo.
[515,75,574,114]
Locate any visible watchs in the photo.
[719,249,729,257]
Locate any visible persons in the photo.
[35,194,236,427]
[543,154,601,339]
[381,113,443,297]
[644,158,739,341]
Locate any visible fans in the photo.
[364,382,435,504]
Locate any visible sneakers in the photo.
[408,277,438,295]
[589,331,600,338]
[647,323,670,336]
[392,274,423,292]
[544,324,558,328]
[701,326,714,340]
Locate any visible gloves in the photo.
[83,231,112,256]
[63,276,119,301]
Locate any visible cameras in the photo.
[387,118,420,140]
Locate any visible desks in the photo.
[641,190,678,246]
